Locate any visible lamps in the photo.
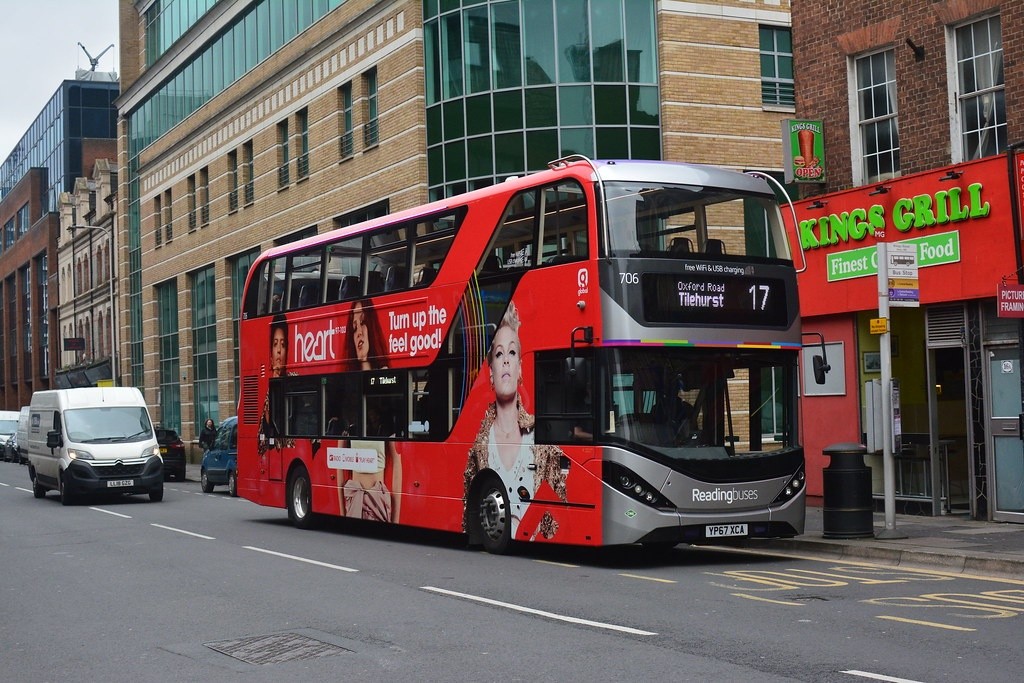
[938,170,964,181]
[806,200,828,210]
[868,186,892,197]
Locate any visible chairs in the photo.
[279,288,298,310]
[483,255,505,272]
[363,271,385,292]
[338,275,358,300]
[320,280,339,302]
[298,284,318,306]
[418,267,438,287]
[385,265,407,291]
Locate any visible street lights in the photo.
[67,224,117,386]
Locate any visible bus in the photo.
[233,155,831,555]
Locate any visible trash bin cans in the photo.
[822,443,875,538]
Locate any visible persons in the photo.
[335,298,402,524]
[257,313,300,456]
[462,300,566,541]
[199,419,217,452]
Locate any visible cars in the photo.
[2,432,17,463]
[156,429,187,481]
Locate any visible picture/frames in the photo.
[891,335,899,357]
[862,351,881,374]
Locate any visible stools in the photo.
[909,456,942,496]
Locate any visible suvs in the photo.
[199,415,237,497]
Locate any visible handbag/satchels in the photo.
[203,442,210,449]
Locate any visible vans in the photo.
[0,410,21,460]
[16,405,29,464]
[27,387,166,506]
